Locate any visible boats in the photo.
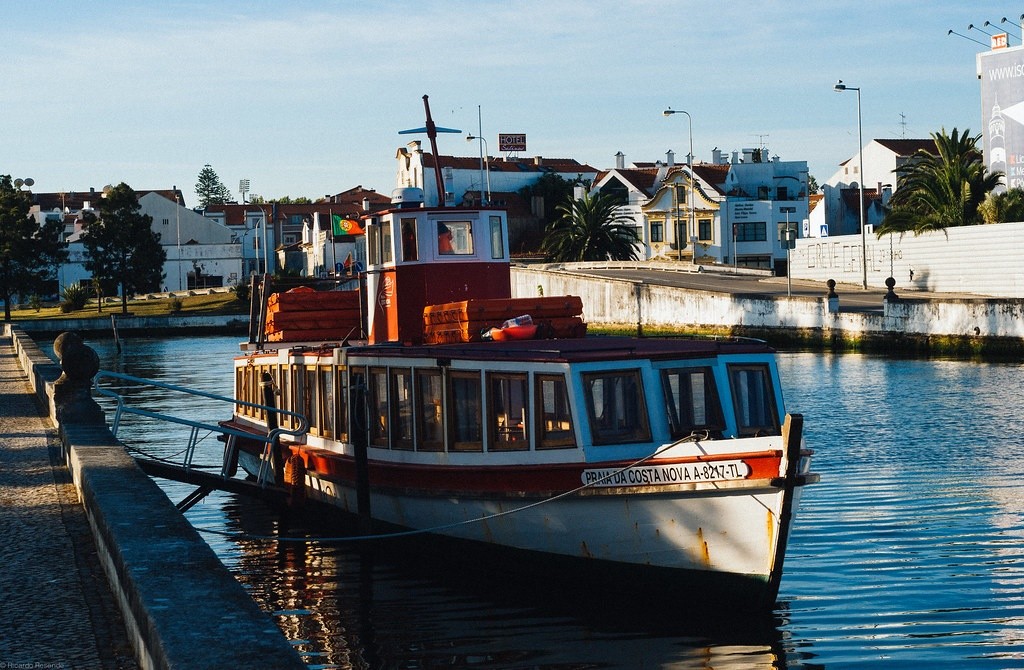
[216,188,816,624]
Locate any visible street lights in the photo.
[467,135,491,201]
[175,194,183,290]
[662,110,697,264]
[834,79,869,290]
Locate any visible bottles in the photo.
[500,315,533,329]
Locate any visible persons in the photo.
[338,271,358,290]
[437,222,455,255]
[402,223,417,261]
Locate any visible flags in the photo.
[336,252,362,273]
[332,214,365,236]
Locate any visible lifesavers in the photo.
[284,454,306,507]
